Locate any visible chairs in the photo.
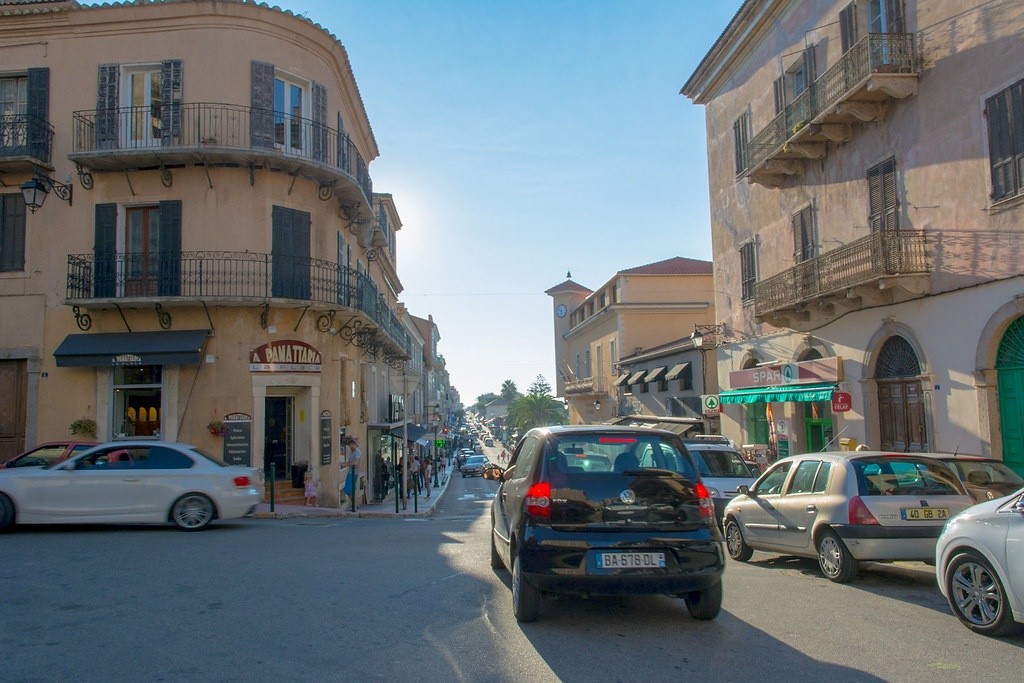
[968,472,993,485]
[616,452,640,473]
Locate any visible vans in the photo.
[638,439,777,529]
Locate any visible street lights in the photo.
[431,417,441,487]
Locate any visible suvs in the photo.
[482,425,726,622]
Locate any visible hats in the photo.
[304,471,313,480]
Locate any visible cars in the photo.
[936,488,1024,637]
[456,415,493,465]
[0,440,262,532]
[460,455,491,478]
[0,440,137,470]
[932,452,1024,505]
[722,450,977,583]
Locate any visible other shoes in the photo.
[306,503,320,507]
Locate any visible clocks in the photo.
[556,304,567,317]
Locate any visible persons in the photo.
[497,447,516,464]
[117,453,148,470]
[379,450,446,500]
[423,457,432,500]
[303,471,320,507]
[340,439,362,511]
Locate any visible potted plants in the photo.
[208,422,225,436]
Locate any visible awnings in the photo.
[643,367,666,382]
[383,423,427,443]
[719,384,839,404]
[665,363,689,381]
[614,374,630,385]
[53,329,210,366]
[627,370,647,385]
[602,417,624,425]
[652,422,695,435]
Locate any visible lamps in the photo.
[594,400,601,409]
[690,322,727,350]
[19,172,72,213]
[564,399,568,409]
